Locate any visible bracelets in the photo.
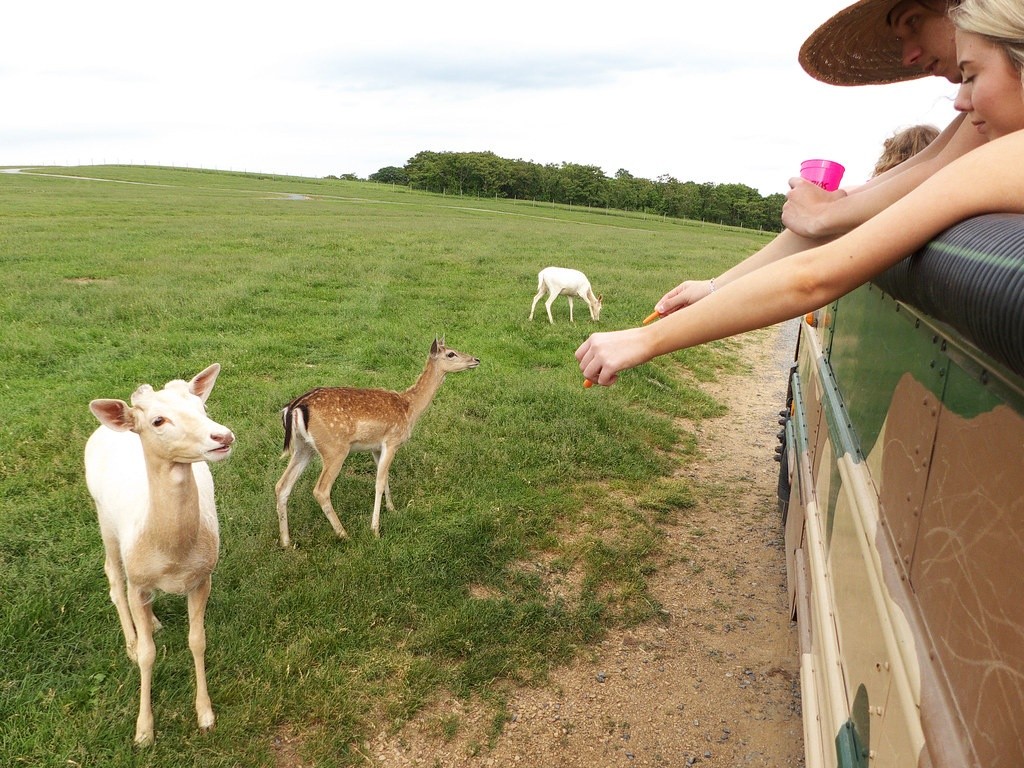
[709,277,715,294]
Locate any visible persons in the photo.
[869,124,939,181]
[655,0,994,320]
[572,0,1022,387]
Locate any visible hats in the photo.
[798,0,935,86]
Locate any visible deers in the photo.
[84,362,236,746]
[529,266,603,326]
[274,333,482,550]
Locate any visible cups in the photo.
[799,158,845,192]
